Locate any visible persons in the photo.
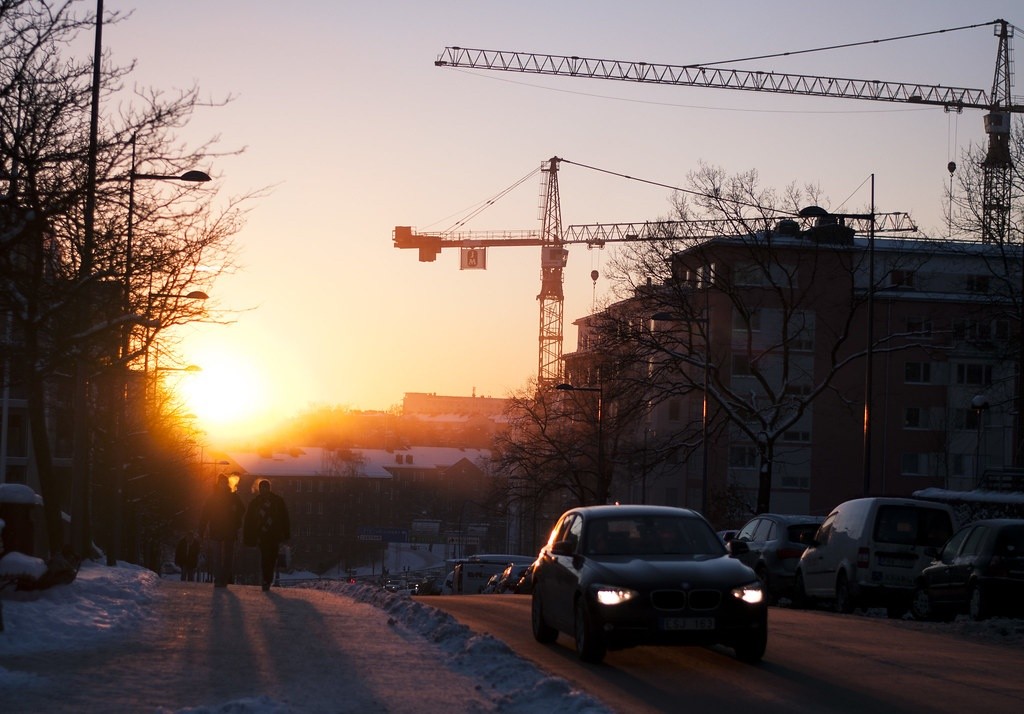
[173,530,199,580]
[242,481,292,593]
[127,525,164,575]
[199,472,244,586]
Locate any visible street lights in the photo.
[650,312,712,518]
[153,364,202,414]
[557,384,608,504]
[798,204,876,497]
[970,394,988,490]
[119,169,212,382]
[142,291,210,420]
[154,413,196,428]
[201,461,230,470]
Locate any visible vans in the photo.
[794,498,960,623]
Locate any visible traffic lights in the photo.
[613,497,623,505]
[349,578,361,583]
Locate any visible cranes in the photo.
[432,19,1024,245]
[391,155,918,426]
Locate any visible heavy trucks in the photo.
[452,555,538,595]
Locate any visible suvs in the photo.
[729,513,829,609]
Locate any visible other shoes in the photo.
[262,581,269,591]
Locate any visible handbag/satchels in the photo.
[277,552,287,573]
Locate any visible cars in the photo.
[913,467,1024,523]
[716,530,740,548]
[483,563,530,595]
[530,501,767,670]
[397,582,419,595]
[382,579,407,592]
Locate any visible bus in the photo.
[914,519,1024,621]
